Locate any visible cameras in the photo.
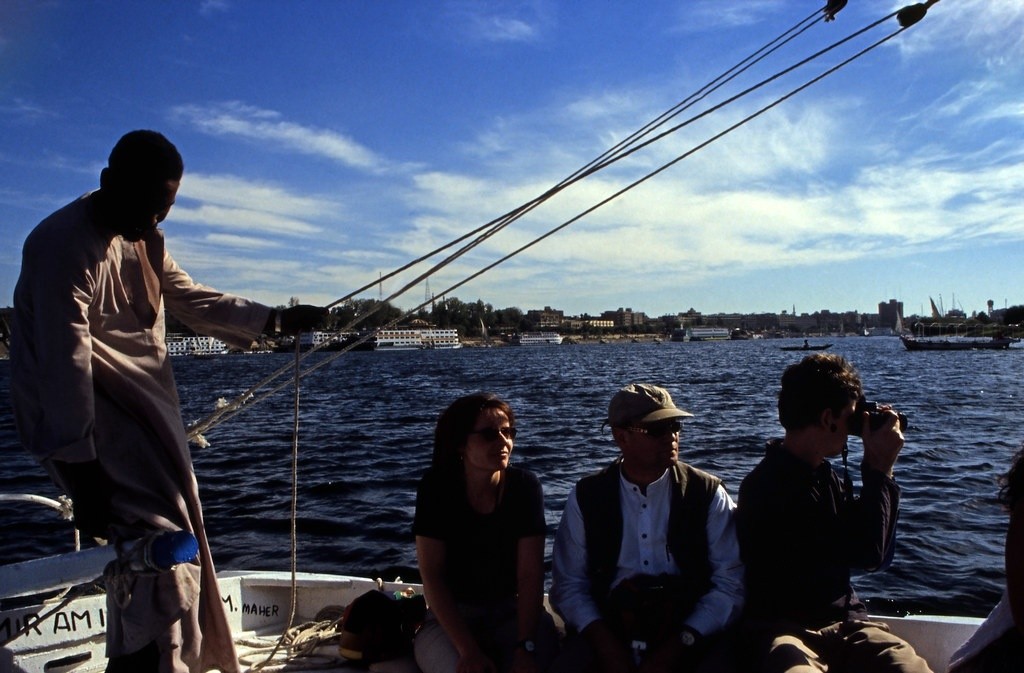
[846,397,908,436]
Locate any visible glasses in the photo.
[469,426,518,442]
[622,421,682,438]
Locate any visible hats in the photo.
[608,383,693,426]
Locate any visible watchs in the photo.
[513,639,537,656]
[679,631,696,650]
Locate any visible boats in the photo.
[860,328,896,336]
[670,327,732,341]
[780,343,835,350]
[516,331,566,346]
[372,329,462,350]
[899,334,1020,350]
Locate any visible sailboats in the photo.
[479,319,491,348]
[187,333,245,358]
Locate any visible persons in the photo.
[10,131,331,673]
[413,394,558,673]
[545,384,751,673]
[947,445,1024,673]
[803,340,808,348]
[734,353,934,672]
